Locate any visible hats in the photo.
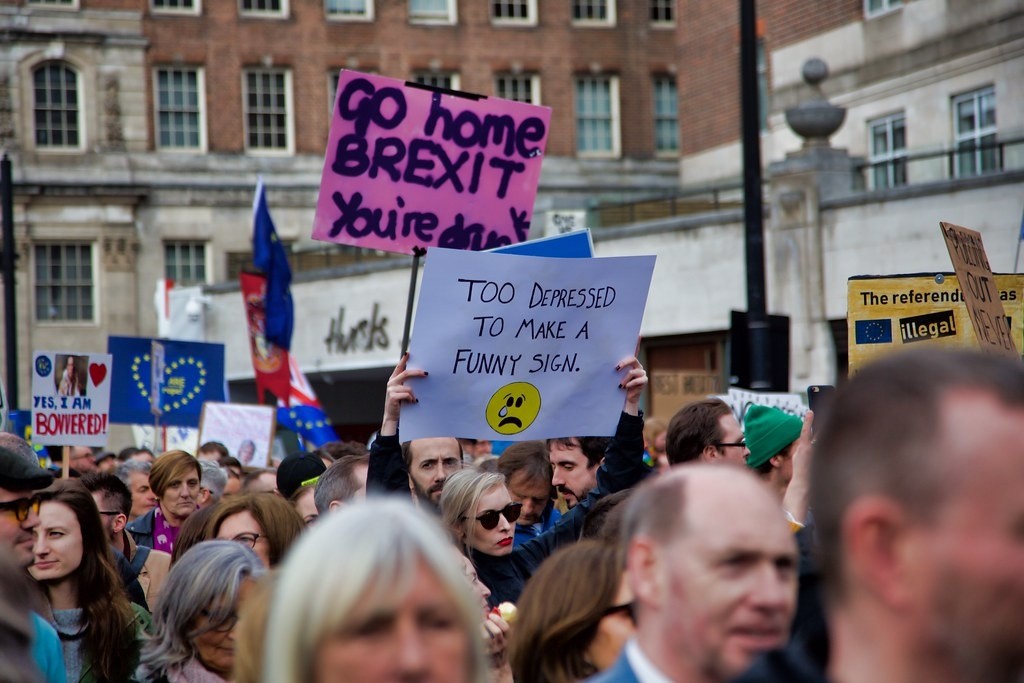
[95,452,115,466]
[0,431,54,491]
[744,405,803,468]
[276,452,327,501]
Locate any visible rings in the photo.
[811,440,817,446]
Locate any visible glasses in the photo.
[698,437,746,455]
[600,602,638,628]
[201,609,239,632]
[71,453,94,461]
[0,495,41,521]
[232,532,268,551]
[461,502,523,530]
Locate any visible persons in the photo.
[262,495,485,683]
[237,440,256,467]
[743,343,1024,683]
[0,399,819,683]
[58,357,85,397]
[367,352,649,610]
[587,463,799,683]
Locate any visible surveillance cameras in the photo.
[186,303,202,322]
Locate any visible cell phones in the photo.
[807,385,835,434]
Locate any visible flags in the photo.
[239,182,339,448]
